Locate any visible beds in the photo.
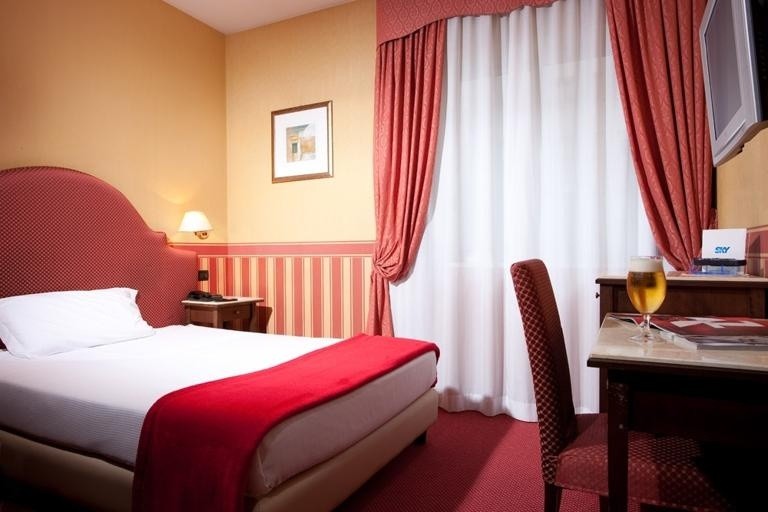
[0,167,440,512]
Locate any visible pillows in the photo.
[1,286,155,359]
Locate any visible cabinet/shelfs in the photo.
[595,272,768,512]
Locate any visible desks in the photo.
[587,313,768,512]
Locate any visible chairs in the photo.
[511,259,717,512]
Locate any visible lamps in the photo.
[177,211,215,241]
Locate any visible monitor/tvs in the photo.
[698,0,768,168]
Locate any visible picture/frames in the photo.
[271,100,333,184]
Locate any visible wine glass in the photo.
[625,256,668,346]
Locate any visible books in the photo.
[607,311,768,351]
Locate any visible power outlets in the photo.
[199,270,209,281]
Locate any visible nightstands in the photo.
[181,296,264,332]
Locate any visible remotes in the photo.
[694,258,747,267]
